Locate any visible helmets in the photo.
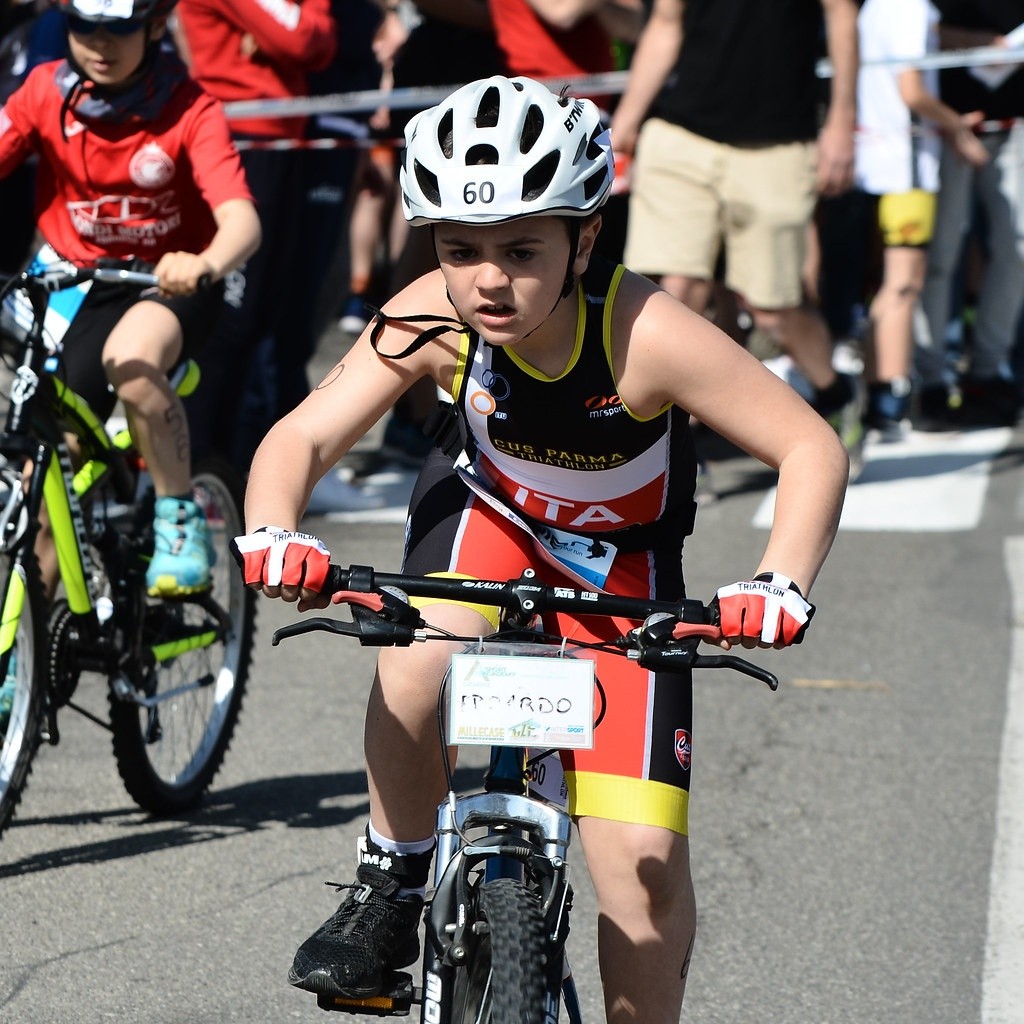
[398,75,616,227]
[54,0,182,36]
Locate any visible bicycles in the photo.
[270,563,805,1022]
[0,267,260,837]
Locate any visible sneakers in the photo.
[691,374,912,509]
[287,864,425,1000]
[144,497,220,598]
[1,641,18,716]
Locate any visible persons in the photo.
[229,75,852,1024]
[919,2,1023,433]
[848,0,991,439]
[0,0,265,714]
[493,0,649,127]
[164,0,394,518]
[607,0,869,506]
[339,0,419,338]
